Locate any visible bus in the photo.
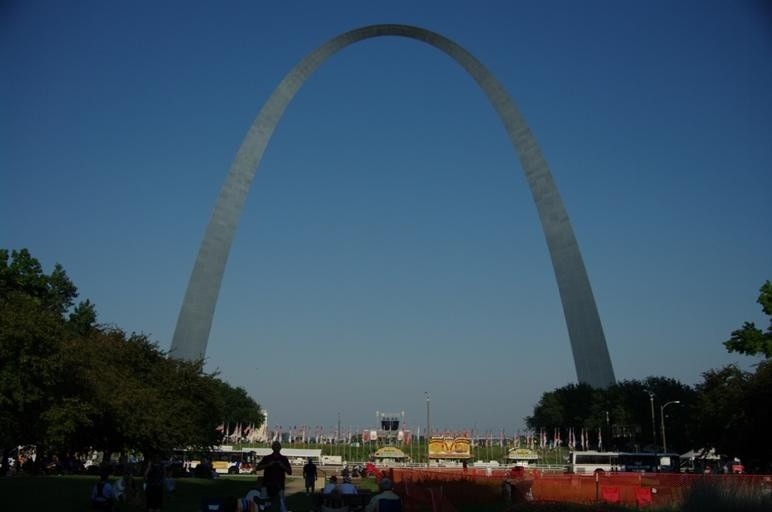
[173,449,256,475]
[567,451,682,480]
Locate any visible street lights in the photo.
[660,400,680,455]
[424,391,432,442]
[642,389,657,453]
[372,409,409,453]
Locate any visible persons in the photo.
[303,457,318,498]
[91,456,537,512]
[255,442,293,510]
[0,451,86,478]
[462,460,470,471]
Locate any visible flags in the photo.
[214,422,604,451]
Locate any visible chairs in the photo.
[600,475,691,509]
[201,478,407,512]
